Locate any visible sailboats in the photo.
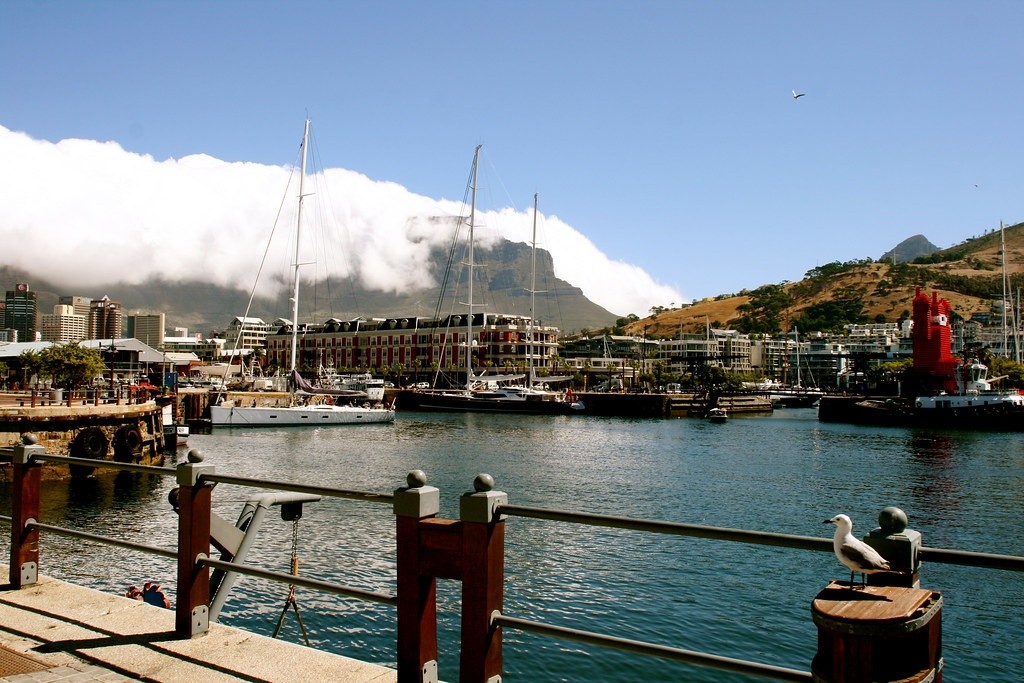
[915,220,1024,417]
[744,307,827,409]
[207,120,397,428]
[414,144,586,415]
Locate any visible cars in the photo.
[416,382,430,389]
[380,383,394,388]
[534,382,550,391]
[606,385,619,392]
[627,384,643,393]
[593,385,608,392]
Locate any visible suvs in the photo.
[84,377,105,390]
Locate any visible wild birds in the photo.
[792,90,805,99]
[822,513,891,591]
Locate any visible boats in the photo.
[158,394,190,446]
[710,408,728,424]
[855,399,916,423]
